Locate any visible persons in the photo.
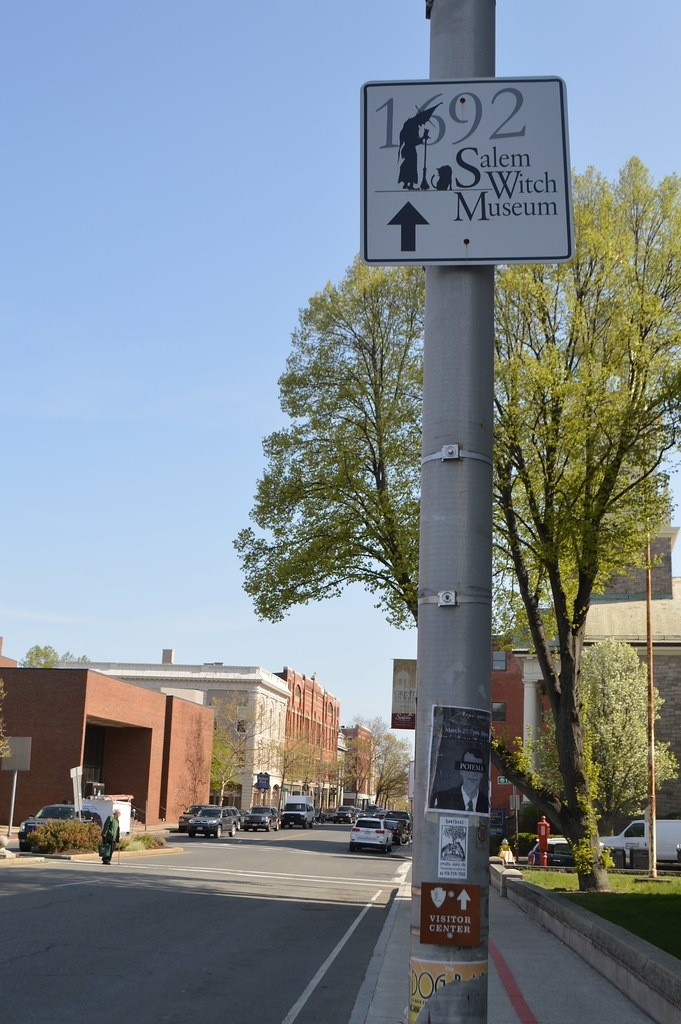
[319,808,324,824]
[101,808,121,865]
[498,838,510,861]
[432,749,489,813]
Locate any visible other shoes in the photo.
[102,858,104,861]
[103,861,110,864]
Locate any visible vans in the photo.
[597,818,681,865]
[282,796,315,829]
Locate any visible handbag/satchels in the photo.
[99,838,111,857]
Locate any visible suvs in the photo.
[179,805,281,839]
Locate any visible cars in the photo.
[18,804,104,853]
[529,837,605,867]
[333,804,411,853]
[350,817,393,854]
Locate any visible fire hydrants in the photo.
[537,816,551,868]
